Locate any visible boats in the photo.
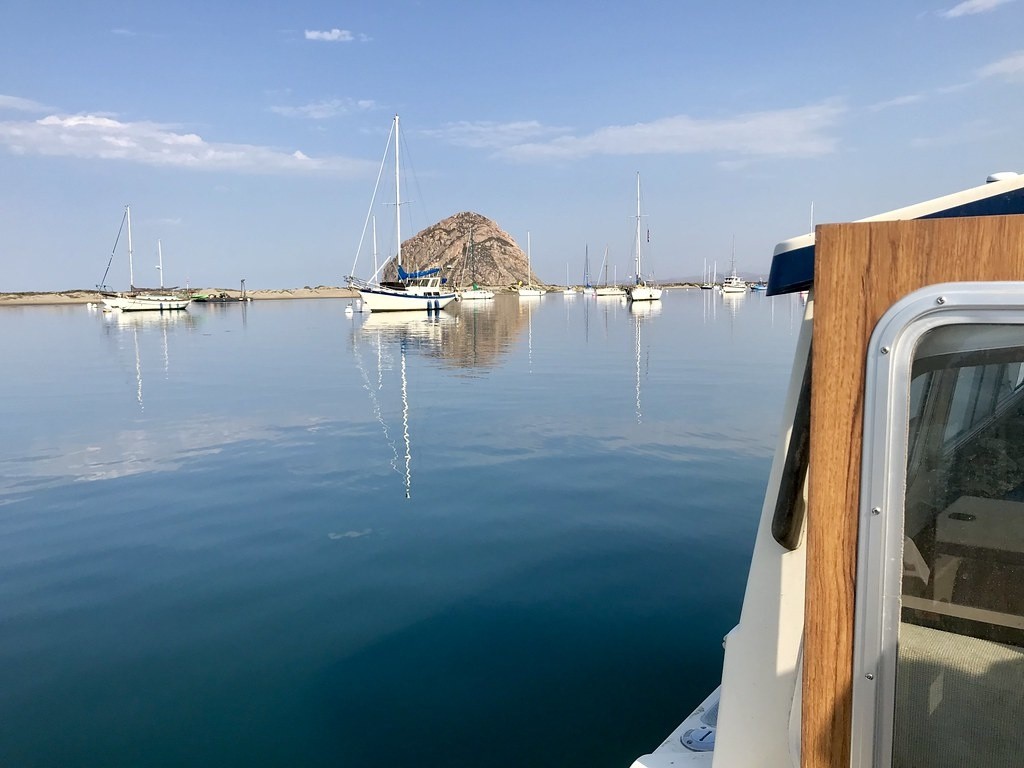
[508,281,527,293]
[190,278,253,302]
[750,277,767,291]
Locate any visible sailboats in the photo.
[580,245,595,295]
[452,225,494,300]
[720,233,748,293]
[116,238,189,312]
[345,112,459,314]
[129,241,185,302]
[511,232,546,296]
[91,203,151,312]
[701,258,717,290]
[561,264,577,295]
[625,170,662,299]
[593,245,626,296]
